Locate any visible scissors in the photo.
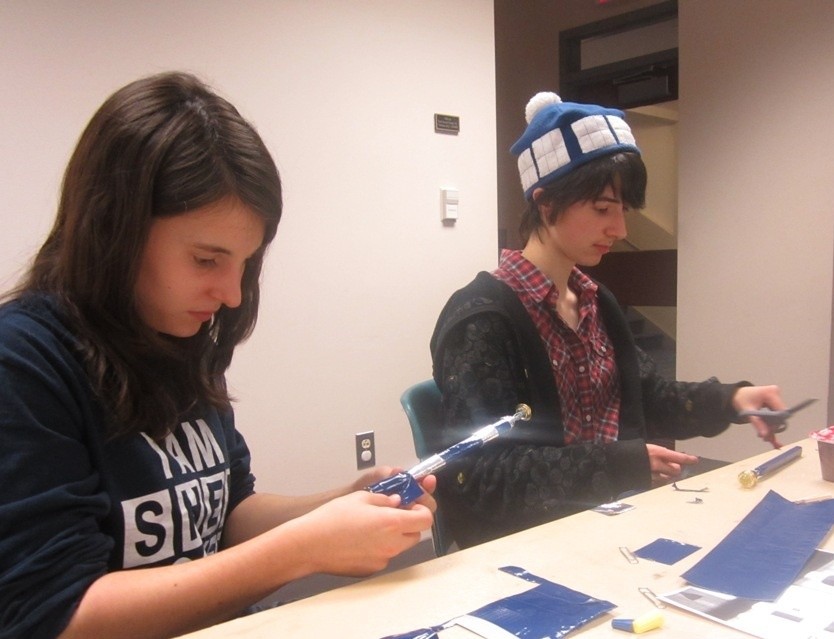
[739,397,818,441]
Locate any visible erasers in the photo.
[611,614,665,635]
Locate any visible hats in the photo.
[509,91,641,201]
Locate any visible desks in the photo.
[170,435,834,639]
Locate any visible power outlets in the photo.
[355,431,376,470]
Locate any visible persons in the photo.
[428,90,789,546]
[0,70,441,639]
